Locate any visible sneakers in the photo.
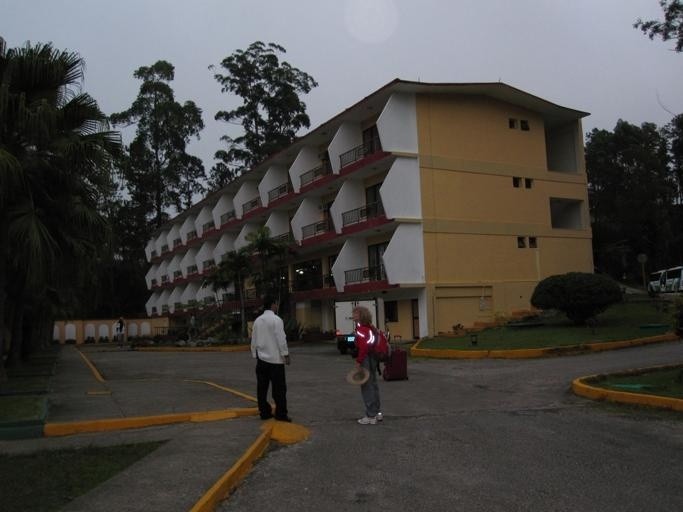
[358,413,383,424]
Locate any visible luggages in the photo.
[383,335,407,381]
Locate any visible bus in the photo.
[647,265,683,294]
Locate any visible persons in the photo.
[250,294,292,422]
[352,305,383,425]
[116,317,125,351]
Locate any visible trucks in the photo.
[333,296,389,354]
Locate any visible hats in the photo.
[347,367,369,385]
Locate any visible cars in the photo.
[173,328,238,346]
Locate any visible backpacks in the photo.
[370,324,391,363]
